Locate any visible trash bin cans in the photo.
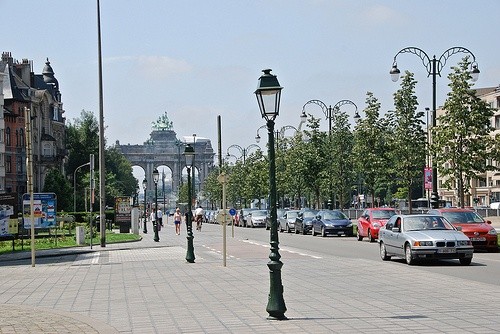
[119,221,130,233]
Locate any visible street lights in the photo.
[253,68,288,320]
[152,167,160,242]
[182,143,197,263]
[142,178,148,233]
[389,45,480,209]
[255,123,300,147]
[224,144,263,166]
[300,99,361,211]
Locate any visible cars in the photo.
[165,208,305,233]
[311,210,353,238]
[356,208,404,244]
[424,209,499,254]
[293,209,323,235]
[377,214,474,266]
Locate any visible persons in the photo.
[194,205,204,230]
[173,207,181,235]
[184,207,193,232]
[156,208,163,226]
[150,209,156,232]
